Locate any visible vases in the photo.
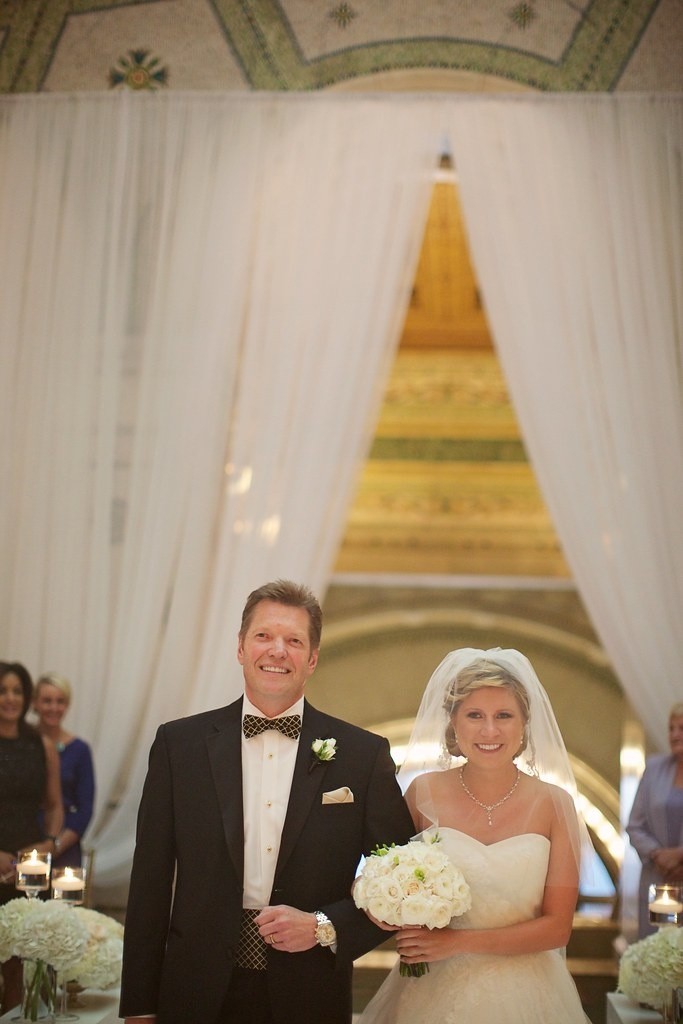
[17,958,59,1024]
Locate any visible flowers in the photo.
[2,898,127,1022]
[615,923,682,1023]
[354,833,472,981]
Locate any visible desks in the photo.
[603,992,682,1023]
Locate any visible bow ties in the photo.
[243,714,302,740]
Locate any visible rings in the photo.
[269,934,276,944]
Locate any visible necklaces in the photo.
[459,761,520,826]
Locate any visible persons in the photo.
[117,579,417,1024]
[357,647,590,1024]
[0,659,64,907]
[623,700,683,942]
[30,671,95,902]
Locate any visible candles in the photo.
[52,866,85,892]
[18,849,49,875]
[649,890,682,916]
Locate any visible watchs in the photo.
[313,909,338,948]
[44,832,62,851]
